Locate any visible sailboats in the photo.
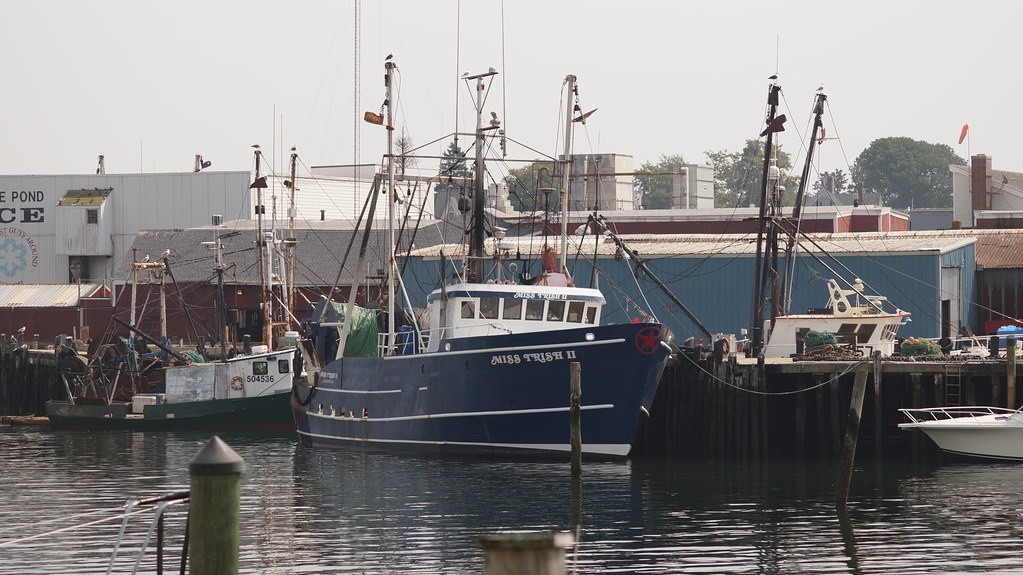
[46,143,313,439]
[311,49,670,465]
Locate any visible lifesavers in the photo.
[231,376,243,391]
[937,338,954,349]
[721,337,730,355]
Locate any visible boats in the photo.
[898,406,1021,461]
